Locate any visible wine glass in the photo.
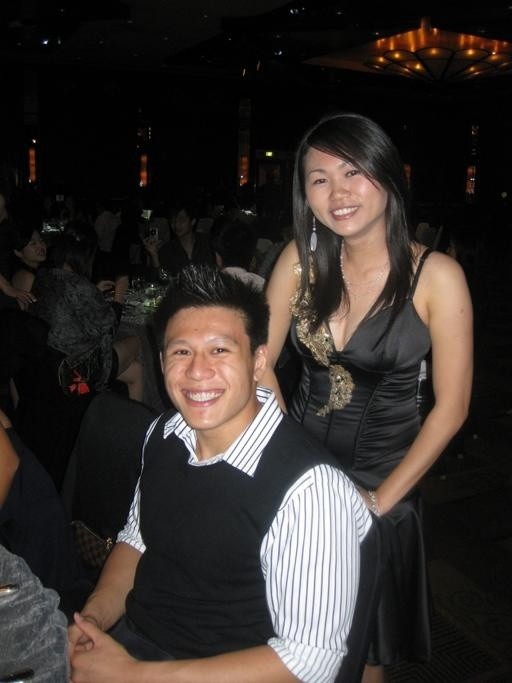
[130,277,160,311]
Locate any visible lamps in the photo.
[359,13,512,83]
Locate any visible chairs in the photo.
[334,508,389,683]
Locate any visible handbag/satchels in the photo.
[65,516,116,573]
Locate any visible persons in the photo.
[2,177,259,614]
[259,110,475,682]
[69,260,384,683]
[416,220,457,259]
